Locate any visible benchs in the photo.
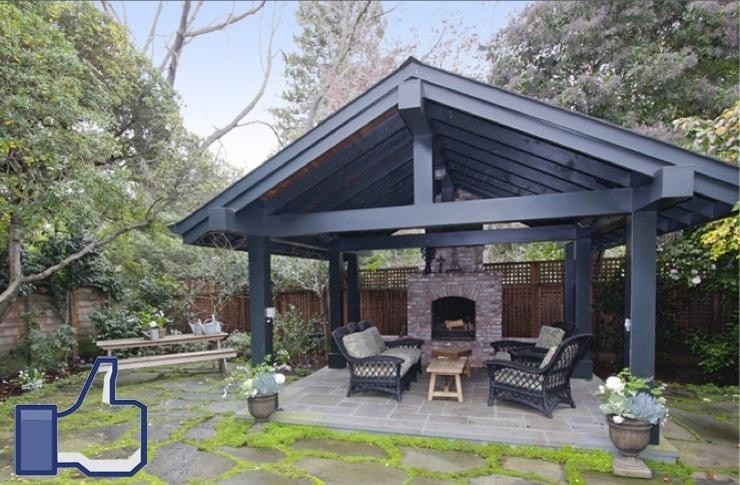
[98,348,238,373]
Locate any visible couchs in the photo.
[331,320,425,402]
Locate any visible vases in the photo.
[247,393,278,422]
[604,413,653,479]
[150,328,158,341]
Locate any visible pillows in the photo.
[539,344,577,372]
[342,326,387,358]
[535,325,566,350]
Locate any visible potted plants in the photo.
[138,304,167,339]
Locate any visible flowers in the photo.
[593,367,672,427]
[149,320,158,327]
[223,349,293,398]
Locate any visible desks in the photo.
[96,332,230,356]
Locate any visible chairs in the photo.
[482,334,594,418]
[490,320,577,368]
